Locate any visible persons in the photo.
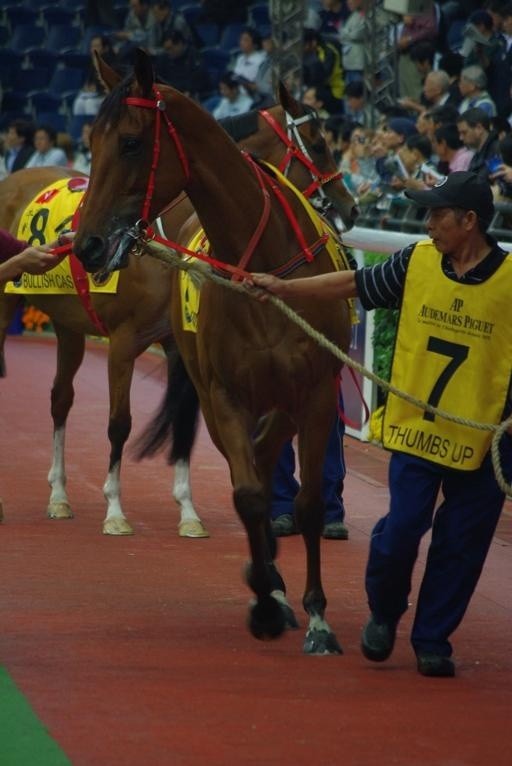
[1,227,79,382]
[263,377,350,540]
[0,0,512,208]
[240,169,511,677]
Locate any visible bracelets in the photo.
[58,228,71,245]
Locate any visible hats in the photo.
[403,169,496,211]
[389,118,418,137]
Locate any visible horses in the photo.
[1,72,359,539]
[70,47,354,657]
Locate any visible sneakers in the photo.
[415,656,456,679]
[360,612,401,663]
[321,520,350,542]
[268,513,305,537]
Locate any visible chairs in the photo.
[1,0,269,141]
